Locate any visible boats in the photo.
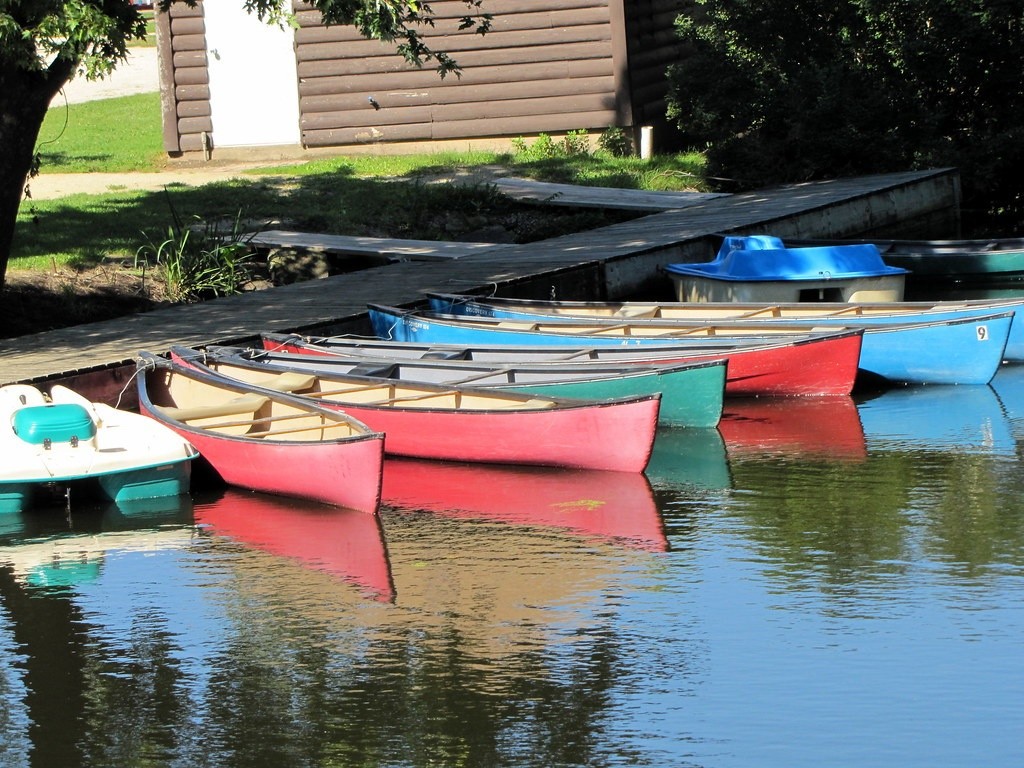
[172,344,660,475]
[662,236,902,304]
[643,426,736,492]
[197,482,396,602]
[854,385,1015,460]
[132,351,384,515]
[0,382,200,538]
[720,388,869,463]
[784,233,1024,273]
[991,357,1024,433]
[378,449,670,556]
[261,287,1024,431]
